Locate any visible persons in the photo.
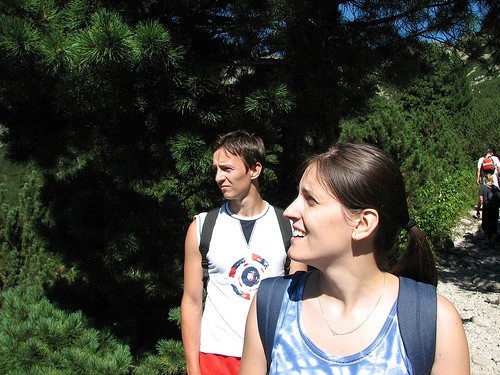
[181,130,307,375]
[479,173,500,249]
[238,143,470,375]
[477,148,500,218]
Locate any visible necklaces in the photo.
[316,275,385,336]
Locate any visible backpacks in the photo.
[481,156,495,173]
[484,184,500,208]
